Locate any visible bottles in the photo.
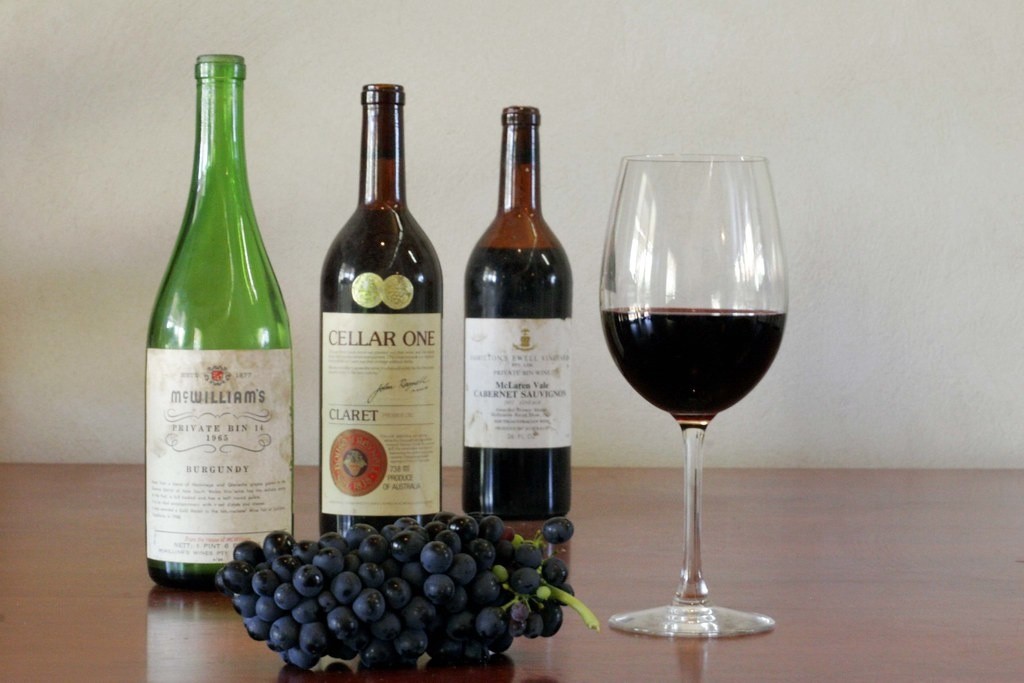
[318,82,443,539]
[145,55,293,590]
[462,106,571,519]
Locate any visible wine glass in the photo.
[597,157,789,640]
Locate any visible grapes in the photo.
[214,507,575,669]
